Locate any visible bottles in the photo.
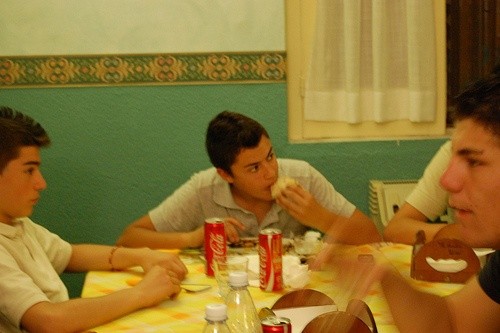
[203,303,231,333]
[222,271,263,333]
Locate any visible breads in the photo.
[270,176,299,198]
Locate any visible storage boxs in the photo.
[369,180,419,240]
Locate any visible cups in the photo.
[295,236,322,260]
[216,256,249,296]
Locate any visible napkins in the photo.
[249,255,310,289]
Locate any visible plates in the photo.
[226,238,294,253]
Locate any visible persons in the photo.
[384,138,458,245]
[333,69,500,333]
[0,106,187,333]
[118,111,381,248]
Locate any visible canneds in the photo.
[258,228,284,292]
[261,316,292,333]
[204,217,228,277]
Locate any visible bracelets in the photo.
[109,246,119,270]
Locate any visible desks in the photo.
[82,248,496,333]
